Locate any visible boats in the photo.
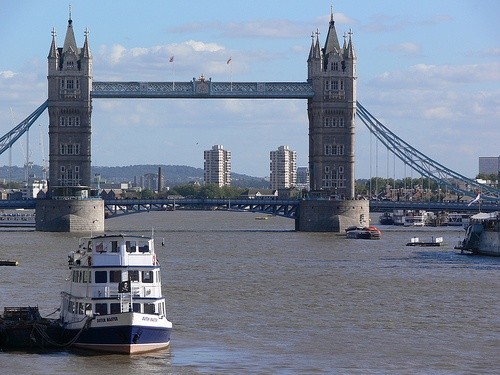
[57,233,173,354]
[453,211,500,257]
[379,212,473,225]
[254,217,268,220]
[1,306,50,347]
[346,226,382,239]
[405,236,443,246]
[0,259,18,266]
